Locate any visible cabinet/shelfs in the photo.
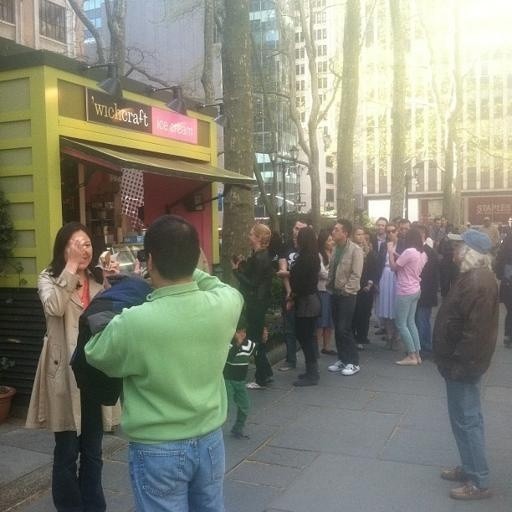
[63,184,121,284]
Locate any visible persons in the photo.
[84,214,244,512]
[432,229,500,499]
[269,216,512,387]
[223,323,258,438]
[25,221,122,512]
[230,223,275,389]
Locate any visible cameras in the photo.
[138,249,150,262]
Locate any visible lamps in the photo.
[200,102,233,129]
[152,84,187,116]
[83,61,124,104]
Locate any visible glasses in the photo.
[388,228,396,235]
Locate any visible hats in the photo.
[446,230,493,254]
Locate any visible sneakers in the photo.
[245,324,422,390]
[450,483,492,500]
[442,466,466,481]
